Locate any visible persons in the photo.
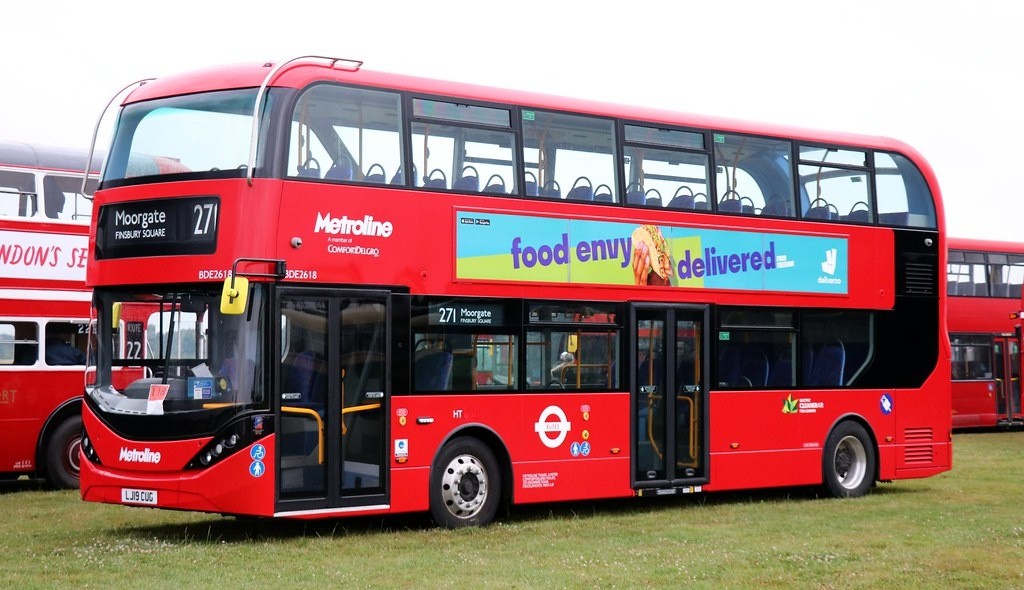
[550,351,575,378]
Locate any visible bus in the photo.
[944,238,1024,430]
[0,141,191,490]
[79,57,955,531]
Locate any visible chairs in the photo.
[639,335,845,388]
[421,166,561,201]
[565,177,614,205]
[295,158,417,187]
[625,181,709,213]
[803,199,871,225]
[947,280,1022,298]
[717,191,790,219]
[414,338,454,391]
